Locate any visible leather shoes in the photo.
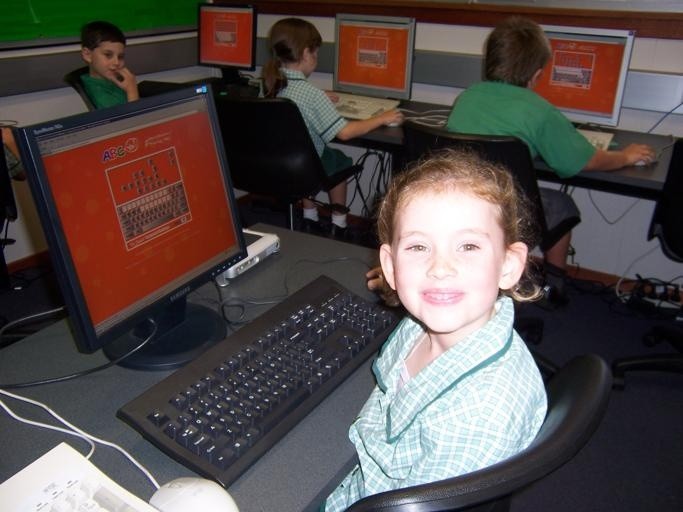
[298,218,353,240]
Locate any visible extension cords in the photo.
[642,295,683,310]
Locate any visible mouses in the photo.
[145,475,238,511]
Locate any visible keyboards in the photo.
[138,80,179,98]
[116,273,402,489]
[577,128,614,153]
[324,89,400,122]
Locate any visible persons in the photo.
[443,14,657,313]
[1,122,28,182]
[317,146,548,512]
[249,17,410,241]
[78,20,141,108]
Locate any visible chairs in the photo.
[394,116,569,348]
[627,134,683,329]
[1,129,54,302]
[63,68,122,126]
[211,85,362,236]
[327,366,595,512]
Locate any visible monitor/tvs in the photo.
[16,82,248,368]
[332,13,416,100]
[530,23,637,125]
[196,4,256,82]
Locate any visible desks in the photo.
[1,226,397,509]
[172,66,683,211]
